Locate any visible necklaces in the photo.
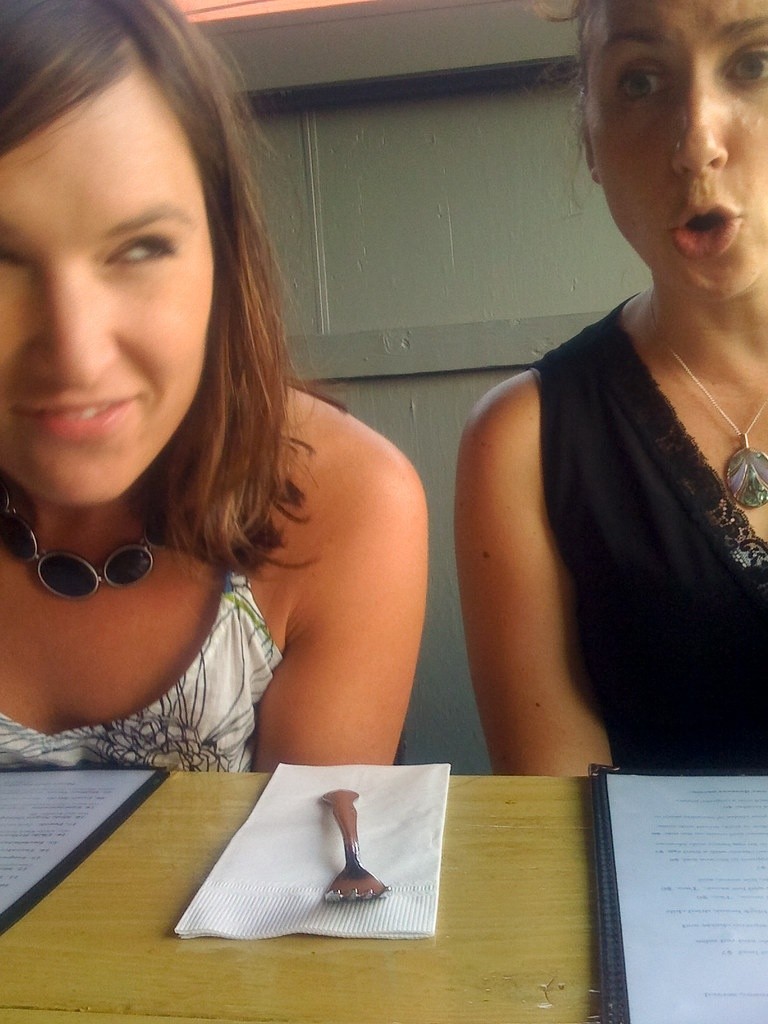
[648,288,767,508]
[0,488,169,600]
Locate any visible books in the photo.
[0,766,171,936]
[588,762,768,1023]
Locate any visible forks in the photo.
[321,789,392,903]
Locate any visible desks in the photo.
[0,765,605,1024]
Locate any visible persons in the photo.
[0,0,428,777]
[454,1,768,777]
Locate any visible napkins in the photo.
[174,763,453,942]
